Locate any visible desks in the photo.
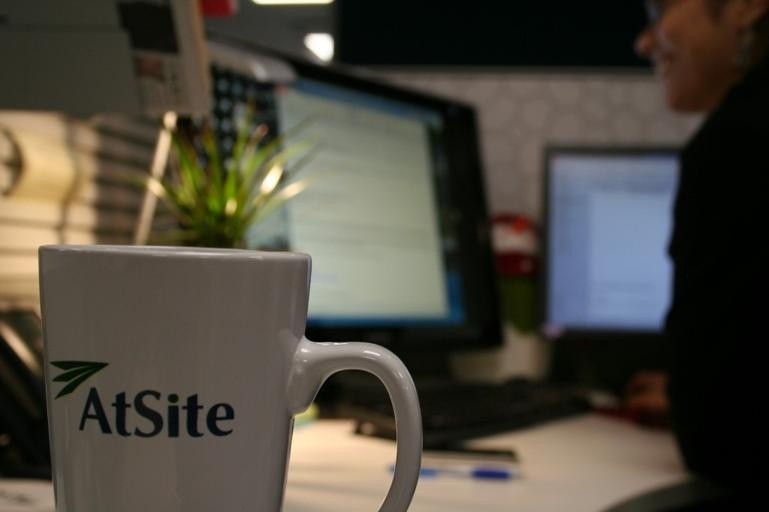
[0,376,715,511]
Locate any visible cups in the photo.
[34,241,426,511]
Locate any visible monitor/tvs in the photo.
[254,53,506,408]
[538,143,684,400]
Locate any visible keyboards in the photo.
[334,376,595,452]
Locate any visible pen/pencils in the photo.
[388,465,509,479]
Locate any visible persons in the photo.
[617,1,769,512]
